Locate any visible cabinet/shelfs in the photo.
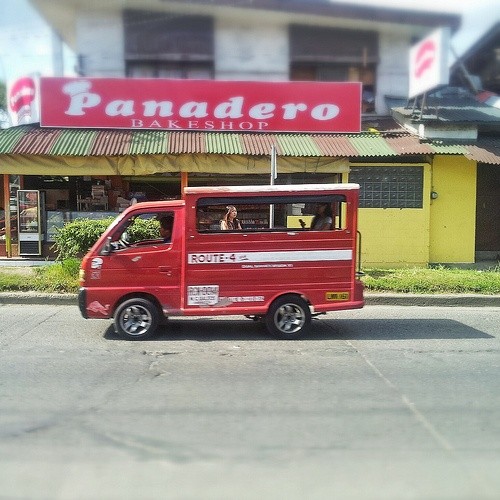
[17,190,47,257]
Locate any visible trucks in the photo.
[77,182,364,339]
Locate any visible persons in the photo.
[300,203,335,229]
[157,216,173,242]
[220,206,244,229]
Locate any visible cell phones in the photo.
[299,219,305,226]
